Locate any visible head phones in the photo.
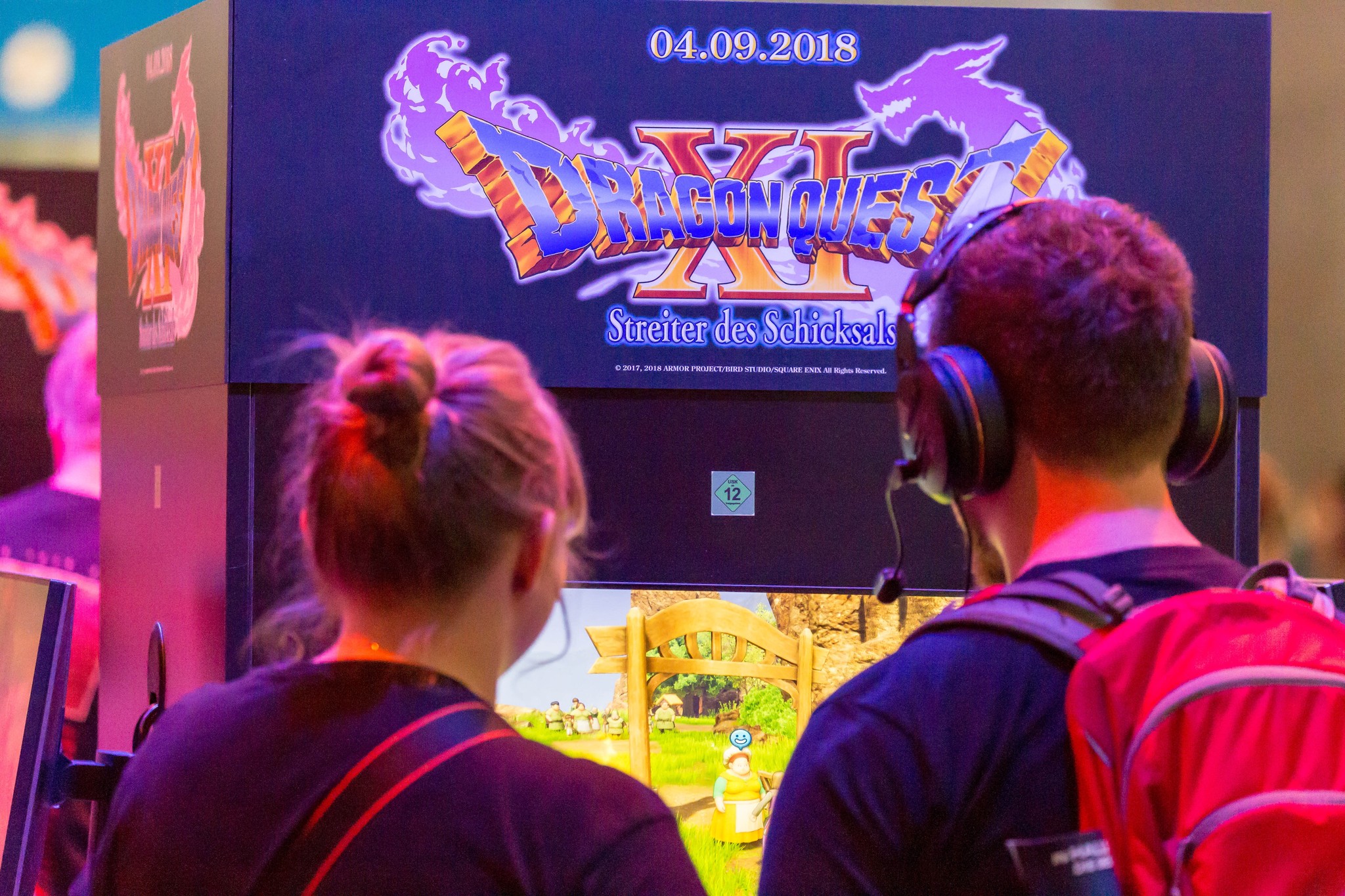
[901,199,1238,503]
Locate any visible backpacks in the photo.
[900,560,1344,896]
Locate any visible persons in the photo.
[71,316,713,896]
[758,194,1265,896]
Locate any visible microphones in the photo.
[873,459,918,603]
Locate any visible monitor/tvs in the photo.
[492,582,979,895]
